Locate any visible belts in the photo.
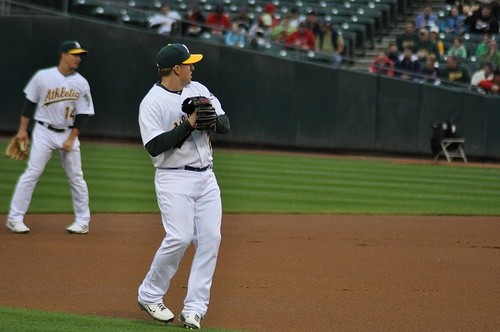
[38,120,75,132]
[156,163,210,172]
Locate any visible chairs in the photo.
[430,121,468,163]
[71,0,500,82]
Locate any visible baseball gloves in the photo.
[6,134,31,161]
[180,96,220,133]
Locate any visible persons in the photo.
[371,0,500,95]
[148,3,346,56]
[139,43,230,330]
[6,39,95,233]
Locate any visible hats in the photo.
[265,4,276,12]
[60,41,89,55]
[153,43,203,64]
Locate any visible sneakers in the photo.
[178,308,201,329]
[67,221,90,234]
[137,301,175,324]
[6,222,31,233]
